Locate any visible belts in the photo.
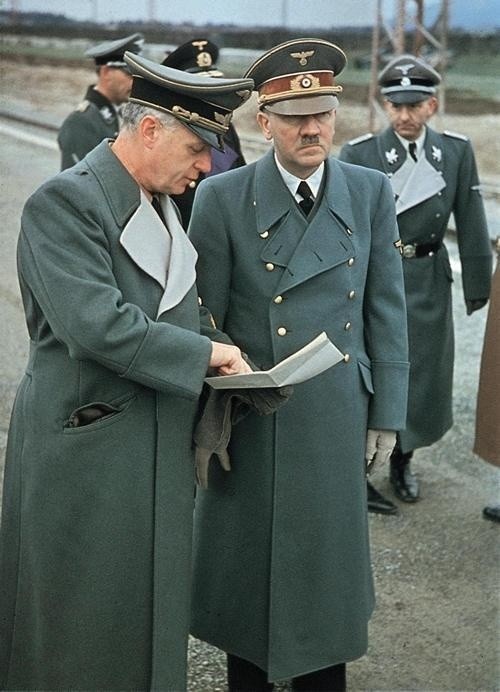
[399,237,442,258]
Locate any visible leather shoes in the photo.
[366,479,398,514]
[485,503,500,521]
[389,462,418,503]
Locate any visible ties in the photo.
[296,180,315,211]
[405,141,419,163]
[150,198,172,228]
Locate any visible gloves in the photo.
[366,429,396,484]
[247,387,295,416]
[195,386,248,487]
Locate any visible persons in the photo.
[337,52,493,515]
[472,236,500,523]
[0,52,294,691]
[183,37,414,691]
[160,38,251,233]
[56,32,145,172]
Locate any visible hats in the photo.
[160,40,220,77]
[85,33,144,77]
[377,56,443,105]
[245,37,348,117]
[122,48,254,153]
